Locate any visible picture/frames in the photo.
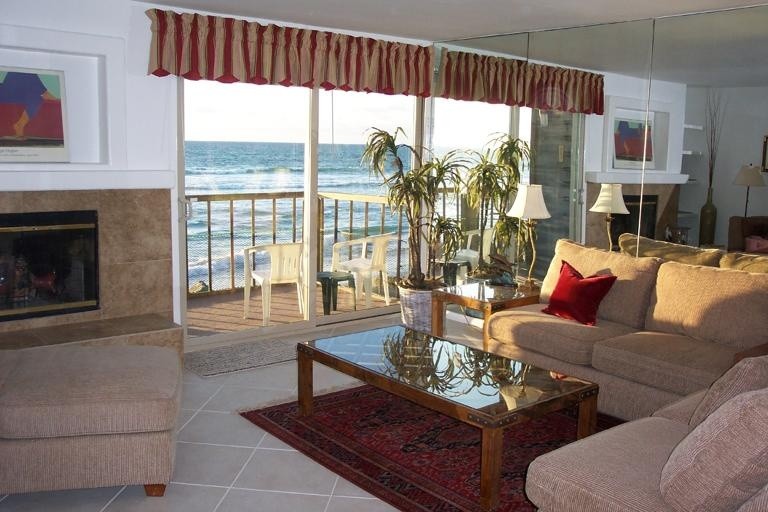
[606,109,656,172]
[0,24,125,172]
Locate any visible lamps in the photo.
[732,163,768,217]
[588,185,630,254]
[505,186,551,293]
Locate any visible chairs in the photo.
[244,244,305,327]
[332,232,393,307]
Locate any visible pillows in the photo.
[489,234,768,511]
[719,253,767,274]
[688,355,767,430]
[541,260,618,327]
[618,234,727,269]
[660,389,768,512]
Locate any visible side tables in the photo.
[431,275,541,353]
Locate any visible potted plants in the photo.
[452,344,534,396]
[698,88,728,247]
[461,132,543,278]
[380,330,462,394]
[360,126,471,335]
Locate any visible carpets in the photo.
[239,385,630,512]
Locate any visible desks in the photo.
[316,272,357,315]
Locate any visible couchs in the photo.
[0,348,180,496]
[619,233,767,273]
